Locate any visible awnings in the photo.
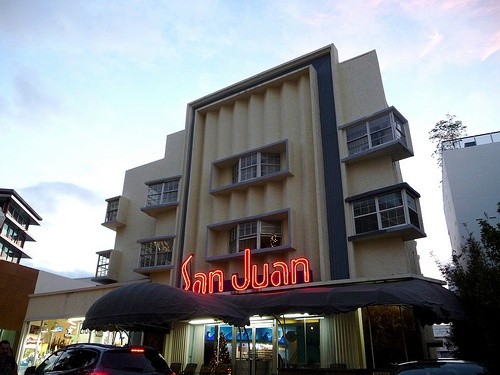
[80,280,478,328]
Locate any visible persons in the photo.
[0,341,20,375]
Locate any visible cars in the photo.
[24,343,175,375]
